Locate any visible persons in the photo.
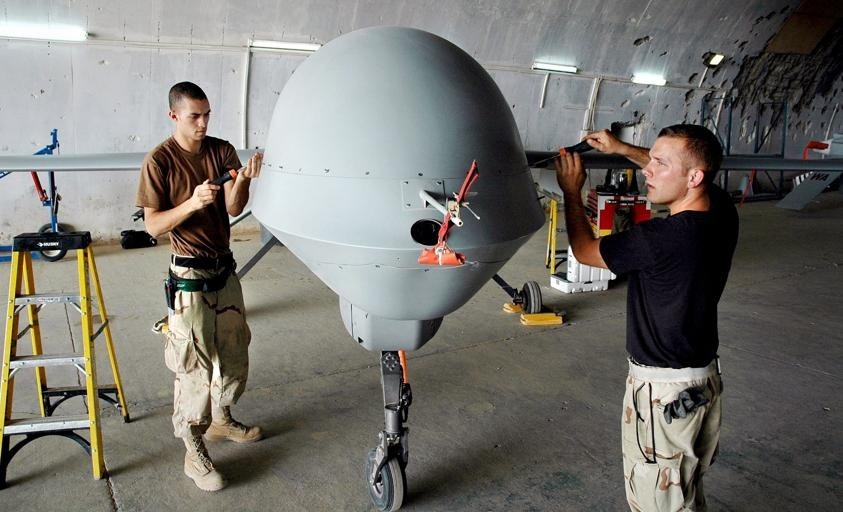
[554,124,739,512]
[136,81,262,493]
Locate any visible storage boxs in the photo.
[547,236,615,295]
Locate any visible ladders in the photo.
[0,231,130,490]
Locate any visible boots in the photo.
[204,404,263,444]
[179,436,229,493]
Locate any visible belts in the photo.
[625,355,719,384]
[170,252,234,268]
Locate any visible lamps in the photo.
[628,73,666,86]
[530,63,578,75]
[702,51,725,69]
[249,40,322,54]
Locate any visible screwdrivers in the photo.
[533,139,597,165]
[210,166,247,185]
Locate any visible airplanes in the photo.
[0,28,843,511]
